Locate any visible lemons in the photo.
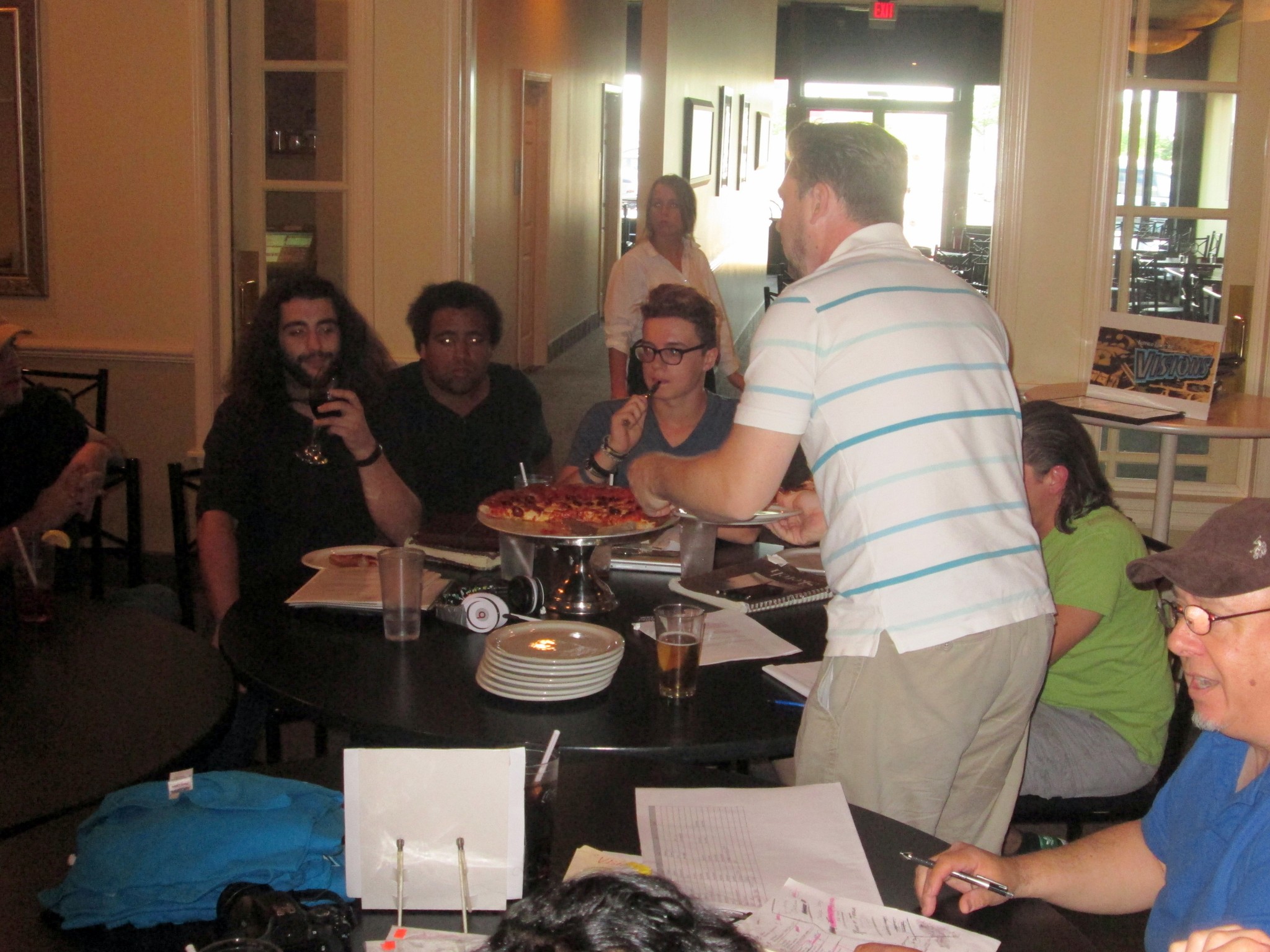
[40,530,70,548]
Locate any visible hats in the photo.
[1126,498,1270,598]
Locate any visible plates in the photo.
[300,545,390,569]
[773,546,826,576]
[474,619,626,702]
[670,505,804,525]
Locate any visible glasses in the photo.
[631,339,705,365]
[1156,598,1270,636]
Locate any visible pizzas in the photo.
[477,484,671,530]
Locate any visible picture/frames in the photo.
[736,95,751,191]
[715,85,733,196]
[683,97,714,189]
[754,112,770,170]
[0,0,48,299]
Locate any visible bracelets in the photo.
[354,441,382,467]
[578,463,600,485]
[589,454,619,477]
[601,434,629,462]
[584,457,608,479]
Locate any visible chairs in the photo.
[763,227,1224,324]
[167,461,330,763]
[1012,534,1194,844]
[19,368,144,604]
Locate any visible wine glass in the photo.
[293,365,351,464]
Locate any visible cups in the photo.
[4,527,58,622]
[270,123,316,155]
[377,547,426,642]
[653,603,706,699]
[498,742,560,882]
[513,473,554,489]
[498,530,536,581]
[196,938,283,952]
[677,517,719,580]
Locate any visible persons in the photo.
[1017,400,1177,797]
[604,174,745,400]
[555,283,741,491]
[626,122,1057,858]
[0,316,126,625]
[914,498,1270,952]
[195,274,397,650]
[379,281,552,516]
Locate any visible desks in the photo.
[1137,257,1224,267]
[1022,383,1269,557]
[1164,267,1223,283]
[0,599,234,840]
[216,526,828,764]
[0,760,1100,952]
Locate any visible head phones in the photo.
[437,575,546,633]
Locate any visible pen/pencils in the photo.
[623,380,661,425]
[767,699,805,707]
[899,852,1014,900]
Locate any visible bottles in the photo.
[1210,255,1217,265]
[1178,253,1188,264]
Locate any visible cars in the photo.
[1112,150,1172,252]
